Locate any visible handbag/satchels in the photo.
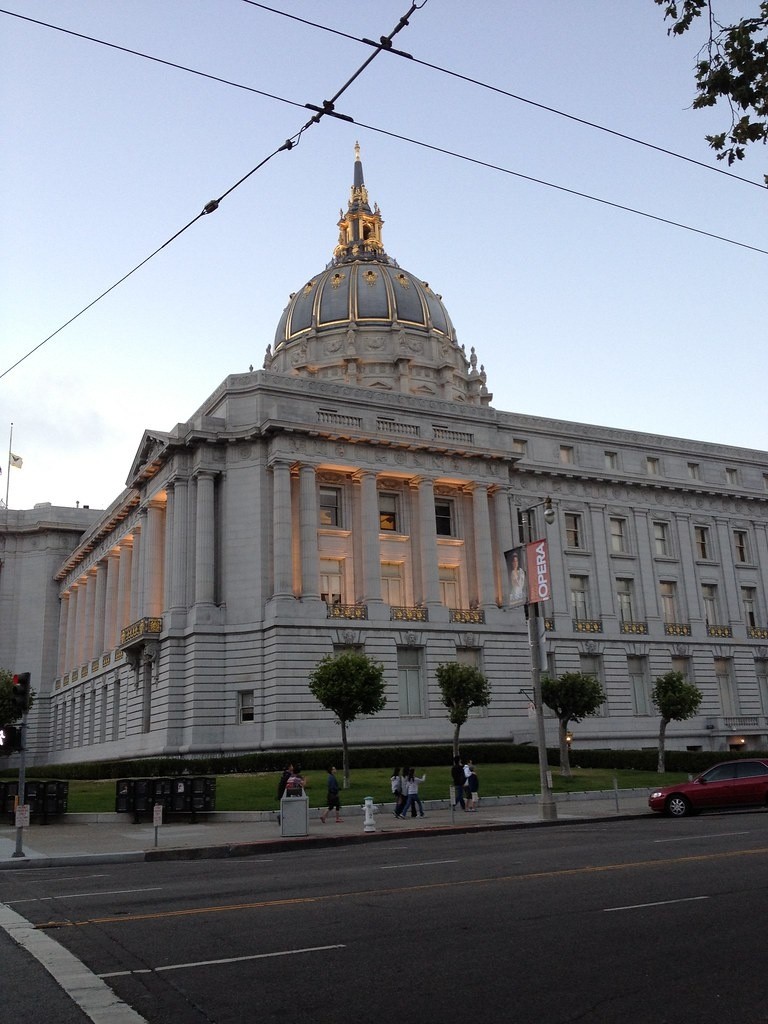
[394,788,401,797]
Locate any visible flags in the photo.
[10,454,22,469]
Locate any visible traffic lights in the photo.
[0,727,16,756]
[9,671,31,712]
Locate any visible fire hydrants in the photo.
[362,796,381,829]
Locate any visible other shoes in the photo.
[452,804,456,811]
[336,818,344,823]
[413,815,419,818]
[465,808,471,812]
[472,809,479,812]
[392,810,397,816]
[398,814,406,819]
[420,814,426,819]
[320,816,325,823]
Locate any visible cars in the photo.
[648,758,768,818]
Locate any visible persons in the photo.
[276,761,307,825]
[319,765,344,823]
[452,756,480,812]
[509,552,524,600]
[391,766,426,819]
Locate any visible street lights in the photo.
[515,496,575,823]
